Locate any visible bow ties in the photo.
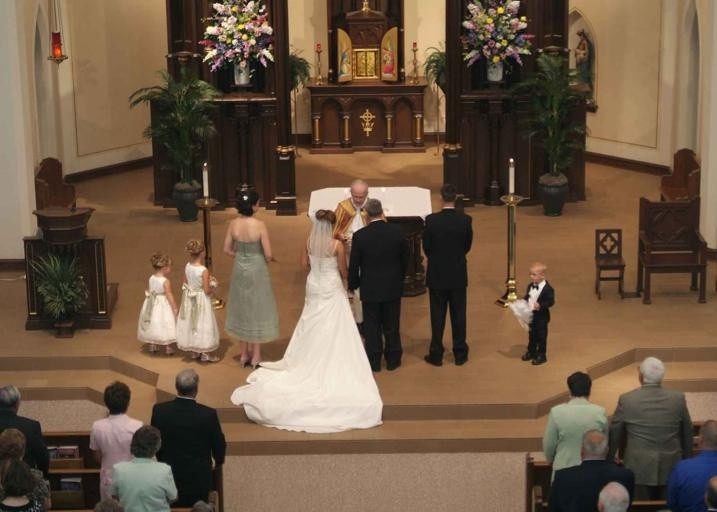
[532,285,539,290]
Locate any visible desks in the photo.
[307,77,428,154]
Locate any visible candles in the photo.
[413,41,417,48]
[316,41,321,50]
[200,161,212,199]
[508,158,517,194]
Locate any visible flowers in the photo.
[196,1,277,73]
[460,0,533,67]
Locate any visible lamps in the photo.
[47,1,69,68]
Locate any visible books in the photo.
[48,446,57,459]
[58,446,80,458]
[60,477,81,490]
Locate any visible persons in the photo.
[335,180,386,334]
[136,252,177,356]
[521,262,554,365]
[542,372,608,487]
[96,499,123,511]
[423,186,472,366]
[224,190,278,368]
[575,48,586,64]
[597,482,629,512]
[230,210,383,433]
[90,381,144,499]
[0,384,49,474]
[570,28,592,92]
[0,427,51,511]
[549,429,634,511]
[109,426,178,511]
[704,475,715,512]
[668,420,717,511]
[609,357,693,502]
[150,370,226,507]
[177,239,221,362]
[349,199,410,371]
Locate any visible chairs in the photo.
[40,430,90,458]
[43,469,100,510]
[594,226,625,297]
[637,196,708,304]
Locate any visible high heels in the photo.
[240,356,250,367]
[251,358,262,368]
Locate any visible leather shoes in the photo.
[165,345,176,355]
[532,353,547,365]
[192,352,200,359]
[200,352,220,362]
[454,355,468,365]
[424,355,442,366]
[522,352,537,361]
[386,360,402,370]
[149,344,160,352]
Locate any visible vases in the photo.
[230,60,250,83]
[485,59,505,81]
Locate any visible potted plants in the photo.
[127,63,222,222]
[510,54,594,215]
[25,250,91,338]
